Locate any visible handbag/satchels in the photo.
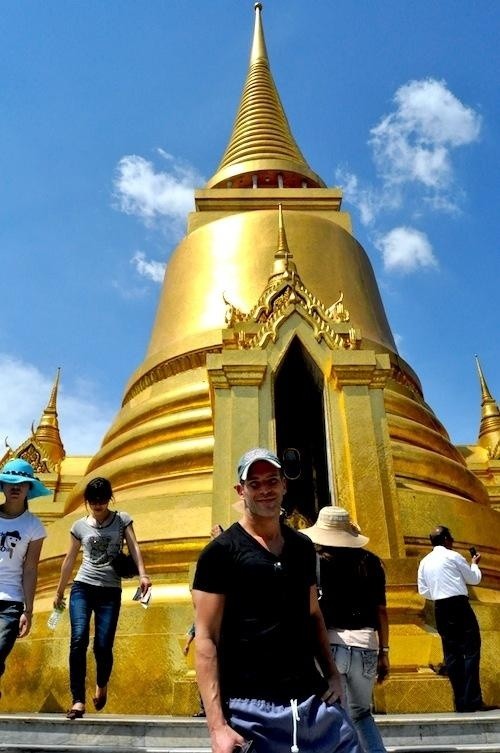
[110,512,140,579]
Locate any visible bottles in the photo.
[47,596,67,629]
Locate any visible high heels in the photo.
[66,700,85,719]
[92,684,107,711]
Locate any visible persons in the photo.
[183,624,207,717]
[210,501,286,539]
[192,449,366,753]
[0,458,54,699]
[417,526,498,713]
[53,476,152,719]
[297,506,390,753]
[209,499,246,540]
[284,509,313,531]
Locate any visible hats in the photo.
[237,448,282,481]
[0,458,53,500]
[297,506,370,548]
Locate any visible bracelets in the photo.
[138,576,150,582]
[379,647,390,652]
[57,594,64,600]
[23,609,33,618]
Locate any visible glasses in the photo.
[87,498,109,505]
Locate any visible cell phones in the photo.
[469,547,477,556]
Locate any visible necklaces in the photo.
[95,510,111,528]
[283,469,302,480]
